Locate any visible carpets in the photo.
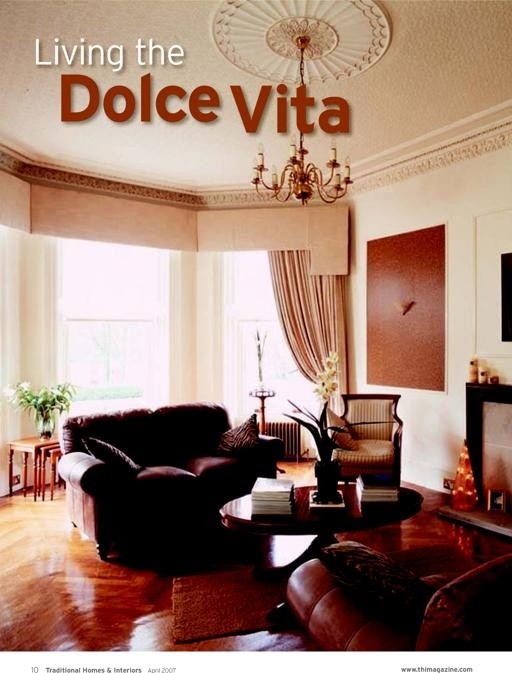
[171,562,284,642]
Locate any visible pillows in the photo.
[327,407,360,451]
[82,435,146,478]
[215,413,260,456]
[320,539,431,632]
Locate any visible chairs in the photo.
[331,393,403,487]
[284,541,512,651]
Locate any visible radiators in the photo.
[257,421,301,463]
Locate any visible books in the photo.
[354,472,400,513]
[251,476,294,515]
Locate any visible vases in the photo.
[311,460,343,504]
[37,417,55,441]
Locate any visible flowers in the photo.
[282,349,397,463]
[2,379,77,437]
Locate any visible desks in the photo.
[8,437,67,501]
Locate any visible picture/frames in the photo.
[487,488,506,513]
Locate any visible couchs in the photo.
[58,401,284,560]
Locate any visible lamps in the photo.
[249,34,354,208]
